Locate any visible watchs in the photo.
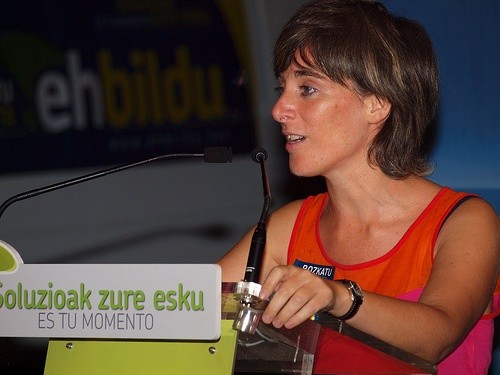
[329,279,364,321]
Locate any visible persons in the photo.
[216,1,500,375]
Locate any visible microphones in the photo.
[0,146,232,218]
[244,148,271,282]
